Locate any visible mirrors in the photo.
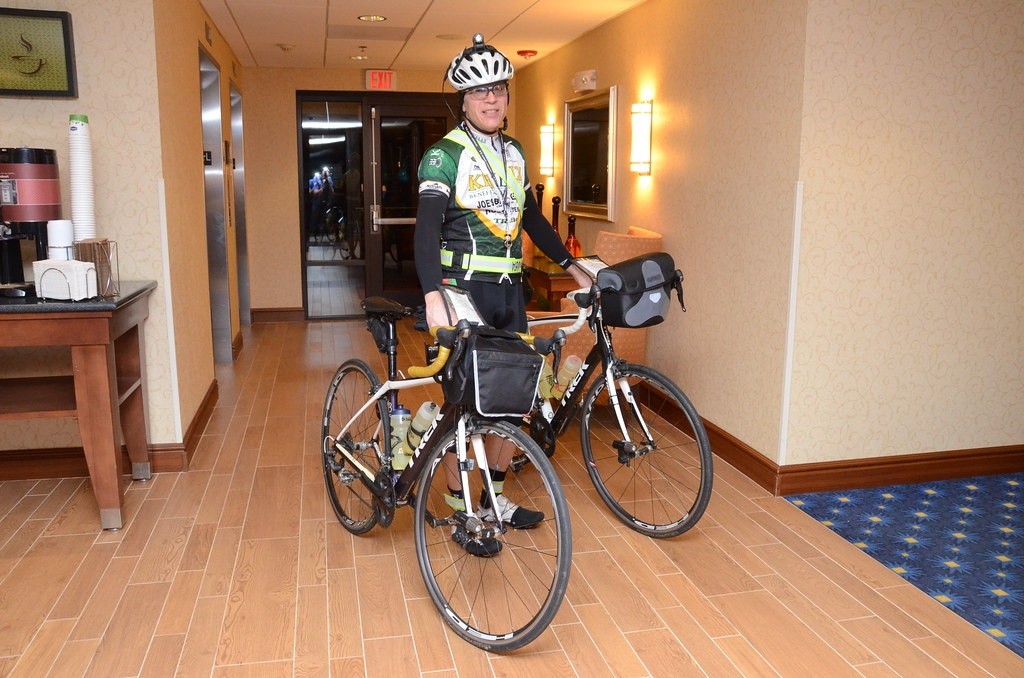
[560,85,619,223]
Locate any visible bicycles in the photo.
[468,252,714,539]
[320,295,574,654]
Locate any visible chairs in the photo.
[525,226,665,425]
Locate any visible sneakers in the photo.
[451,523,502,557]
[476,493,544,527]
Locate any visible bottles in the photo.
[391,404,411,470]
[401,401,440,454]
[539,363,555,398]
[548,196,563,263]
[565,215,582,258]
[551,356,582,399]
[534,183,546,257]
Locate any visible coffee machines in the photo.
[0,147,62,297]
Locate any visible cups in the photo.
[68,114,97,241]
[47,220,74,260]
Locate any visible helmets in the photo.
[447,44,514,90]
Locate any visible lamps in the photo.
[626,96,654,178]
[537,123,555,178]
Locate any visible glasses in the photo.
[460,82,509,100]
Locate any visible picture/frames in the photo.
[0,7,84,101]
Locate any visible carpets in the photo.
[783,472,1024,658]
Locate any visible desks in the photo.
[0,278,158,531]
[524,257,581,314]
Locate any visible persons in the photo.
[418,32,593,558]
[309,152,363,262]
